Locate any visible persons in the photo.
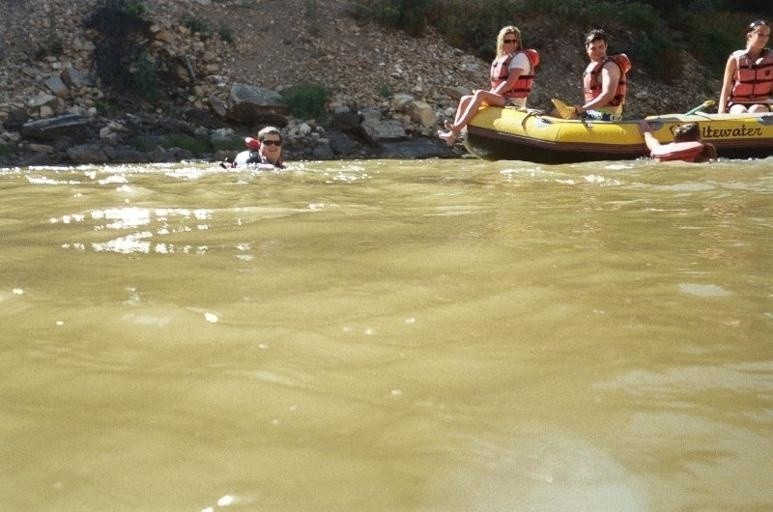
[717,18,772,116]
[437,23,532,147]
[565,28,625,121]
[246,124,296,170]
[670,125,723,161]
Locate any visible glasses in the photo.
[262,140,280,146]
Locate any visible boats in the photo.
[460,100,772,166]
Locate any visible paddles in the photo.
[552,99,577,120]
